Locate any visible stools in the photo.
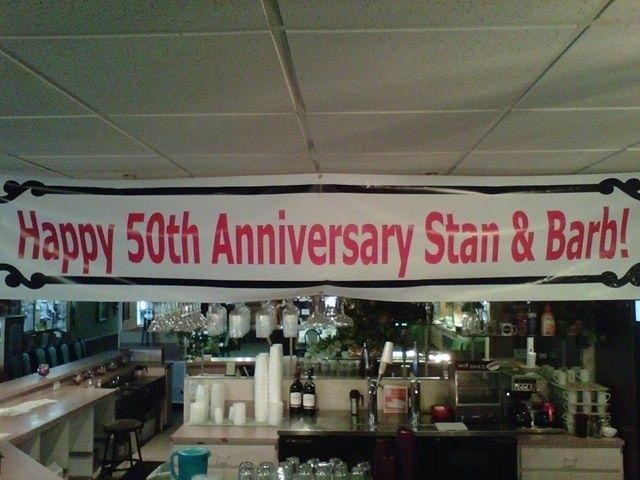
[100,420,145,479]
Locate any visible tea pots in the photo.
[133,364,148,381]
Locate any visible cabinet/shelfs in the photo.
[171,424,277,474]
[519,434,626,480]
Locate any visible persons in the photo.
[343,297,412,376]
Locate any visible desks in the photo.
[0,349,173,480]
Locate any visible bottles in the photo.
[287,361,318,418]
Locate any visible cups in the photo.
[190,383,247,426]
[349,389,364,416]
[499,320,518,337]
[254,344,284,426]
[380,341,394,365]
[572,414,588,438]
[169,447,212,480]
[551,366,611,434]
[238,457,372,480]
[513,300,556,337]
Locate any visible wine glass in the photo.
[36,362,52,381]
[69,355,130,384]
[459,301,488,337]
[139,299,353,341]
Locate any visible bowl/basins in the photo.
[431,404,450,419]
[601,426,618,439]
